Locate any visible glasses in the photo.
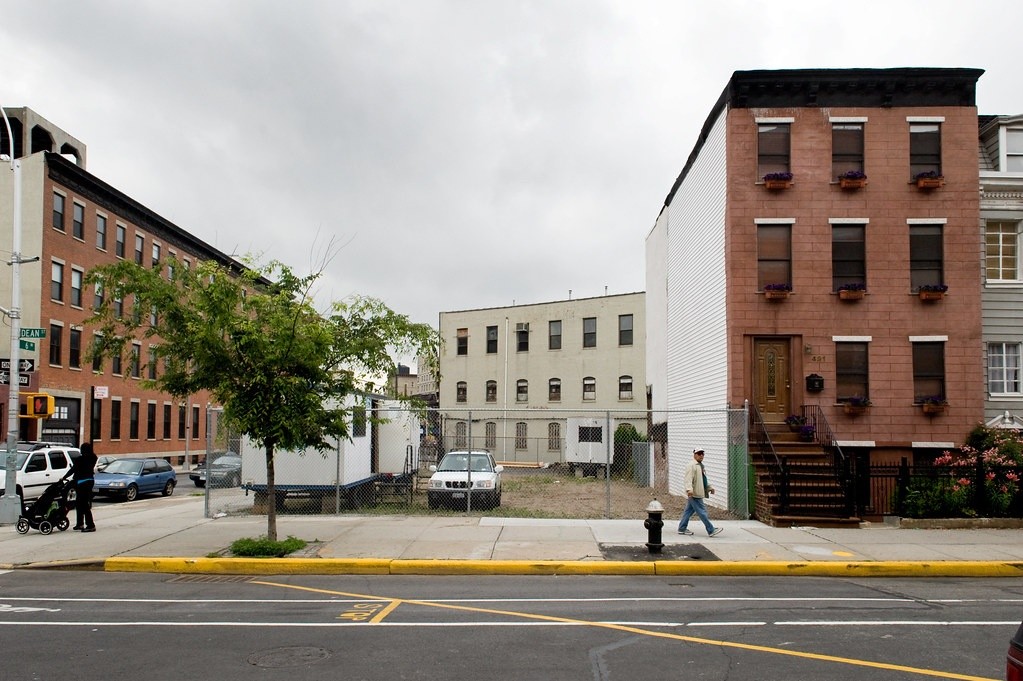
[695,452,705,456]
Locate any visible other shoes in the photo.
[82,527,96,532]
[73,525,84,529]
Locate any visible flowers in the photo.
[840,170,868,180]
[785,415,807,424]
[919,284,949,292]
[917,171,940,180]
[848,397,872,406]
[920,395,942,404]
[838,283,866,291]
[764,172,793,181]
[764,283,792,291]
[803,426,814,431]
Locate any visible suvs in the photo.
[0,440,82,507]
[427,447,504,508]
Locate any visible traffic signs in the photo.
[0,358,35,372]
[19,339,36,352]
[19,328,46,340]
[0,371,31,387]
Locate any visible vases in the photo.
[918,178,942,189]
[790,424,803,432]
[802,432,813,442]
[764,290,787,299]
[844,404,864,415]
[922,404,943,413]
[765,180,790,190]
[919,290,942,300]
[839,179,864,189]
[839,290,865,301]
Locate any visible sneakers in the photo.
[709,528,723,537]
[678,529,694,535]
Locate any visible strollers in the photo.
[15,477,79,535]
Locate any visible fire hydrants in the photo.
[644,495,666,555]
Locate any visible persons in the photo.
[61,443,98,532]
[677,447,723,537]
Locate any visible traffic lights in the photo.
[32,394,55,417]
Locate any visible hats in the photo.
[693,447,705,453]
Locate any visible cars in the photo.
[188,455,243,488]
[92,456,118,478]
[196,451,238,468]
[92,457,179,501]
